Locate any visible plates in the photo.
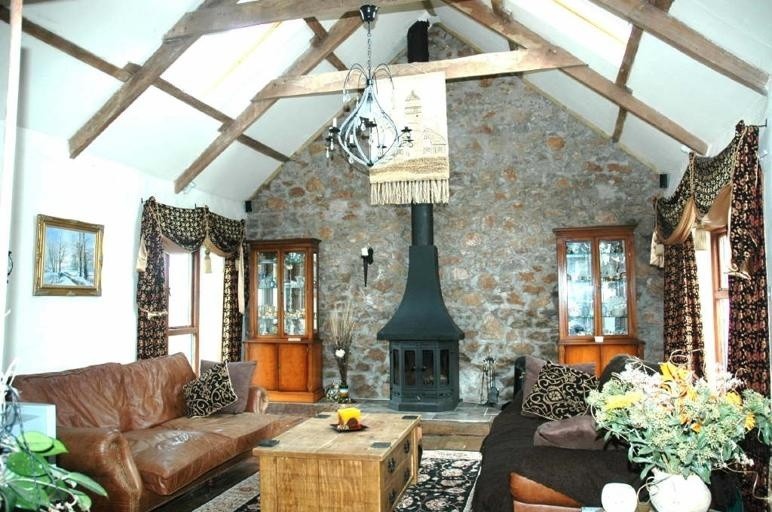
[330,424,369,432]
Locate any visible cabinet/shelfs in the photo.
[241,237,325,404]
[553,221,644,379]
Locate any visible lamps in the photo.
[361,246,373,287]
[325,5,415,176]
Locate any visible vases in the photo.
[326,343,362,402]
[646,465,712,512]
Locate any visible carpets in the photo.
[191,448,483,512]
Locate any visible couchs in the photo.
[472,355,749,511]
[7,352,276,511]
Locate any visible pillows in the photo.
[521,360,596,418]
[200,360,257,414]
[523,355,596,423]
[535,414,607,453]
[183,358,238,420]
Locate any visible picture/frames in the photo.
[33,214,104,297]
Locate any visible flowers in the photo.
[585,356,772,484]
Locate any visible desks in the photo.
[0,403,56,473]
